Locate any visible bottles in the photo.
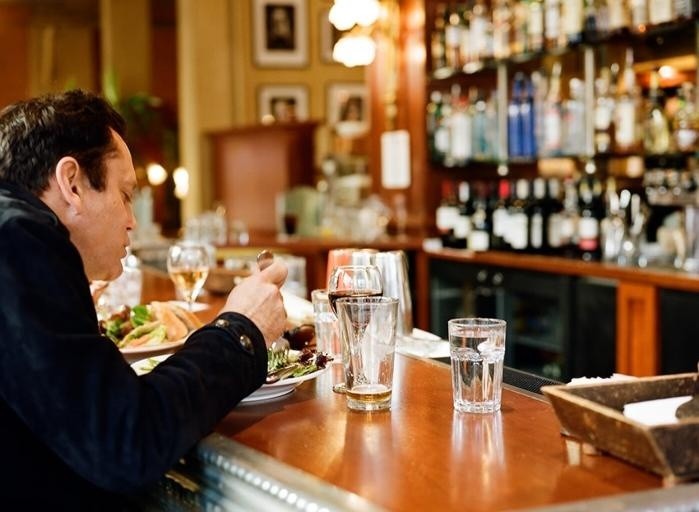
[427,61,699,163]
[426,0,699,80]
[423,168,699,274]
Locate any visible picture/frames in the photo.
[319,9,342,65]
[254,82,311,127]
[249,1,311,70]
[325,81,371,137]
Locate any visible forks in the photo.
[257,249,290,368]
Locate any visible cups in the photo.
[311,289,333,358]
[447,318,507,414]
[335,296,399,413]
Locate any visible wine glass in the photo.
[166,244,210,313]
[328,265,384,394]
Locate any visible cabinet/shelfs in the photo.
[421,0,698,277]
[415,246,631,398]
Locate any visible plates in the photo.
[130,349,334,402]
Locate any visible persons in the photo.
[0,88,290,512]
